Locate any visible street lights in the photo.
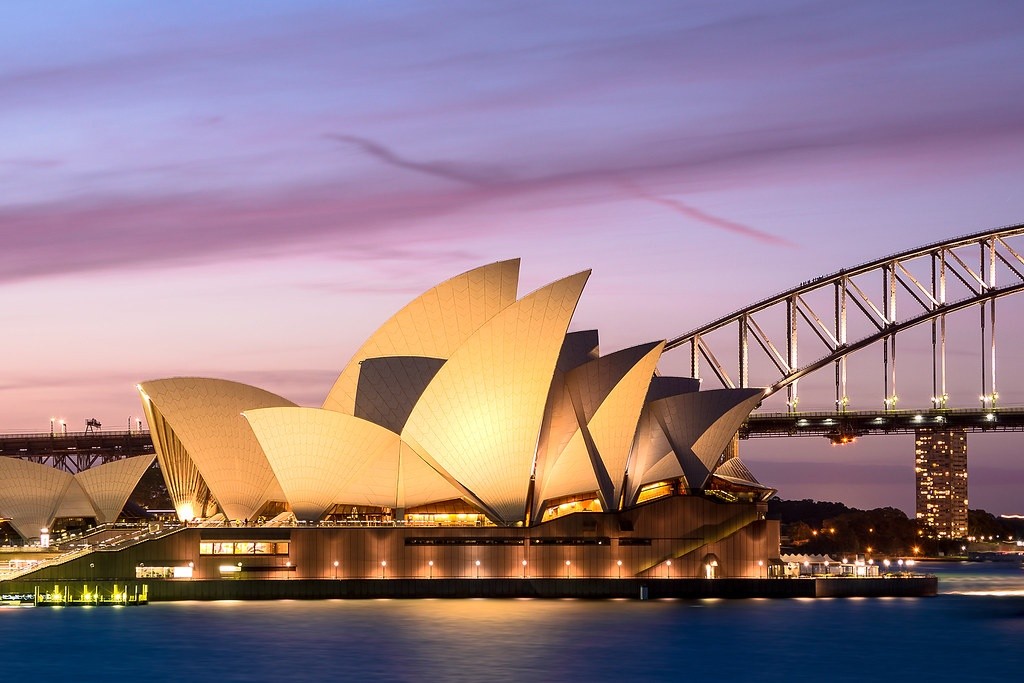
[523,560,528,578]
[429,561,434,578]
[617,560,622,578]
[667,561,671,578]
[759,561,764,579]
[381,561,386,578]
[805,561,809,577]
[566,560,571,579]
[476,561,480,579]
[286,562,290,579]
[334,561,339,579]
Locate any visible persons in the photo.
[159,516,175,522]
[223,516,265,527]
